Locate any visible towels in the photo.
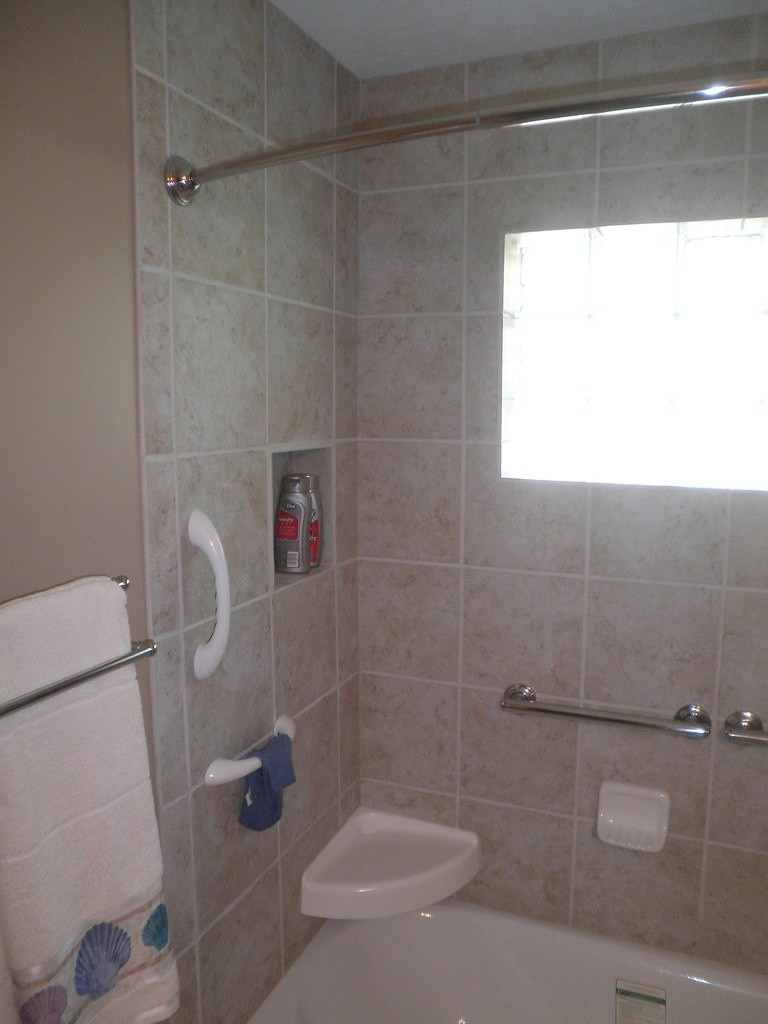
[0,574,182,1024]
[236,735,298,834]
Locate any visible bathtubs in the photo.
[245,895,768,1024]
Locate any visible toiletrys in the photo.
[274,474,314,577]
[297,473,325,568]
